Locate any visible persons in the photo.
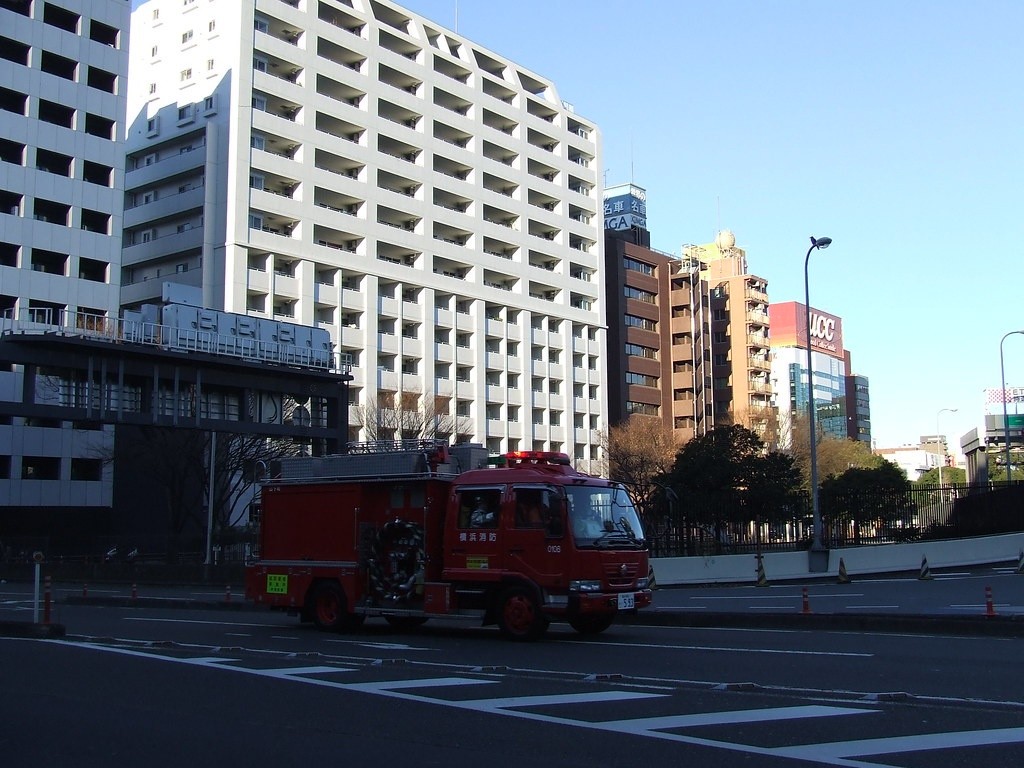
[789,525,794,542]
[470,495,496,528]
[719,525,730,552]
[572,493,593,518]
[527,491,553,528]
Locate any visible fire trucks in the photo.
[245,438,652,642]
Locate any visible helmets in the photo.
[472,494,488,510]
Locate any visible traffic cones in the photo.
[836,558,851,584]
[1015,551,1024,574]
[649,564,660,590]
[755,553,769,587]
[917,554,933,580]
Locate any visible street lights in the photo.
[1001,330,1024,480]
[936,408,958,503]
[805,236,831,549]
